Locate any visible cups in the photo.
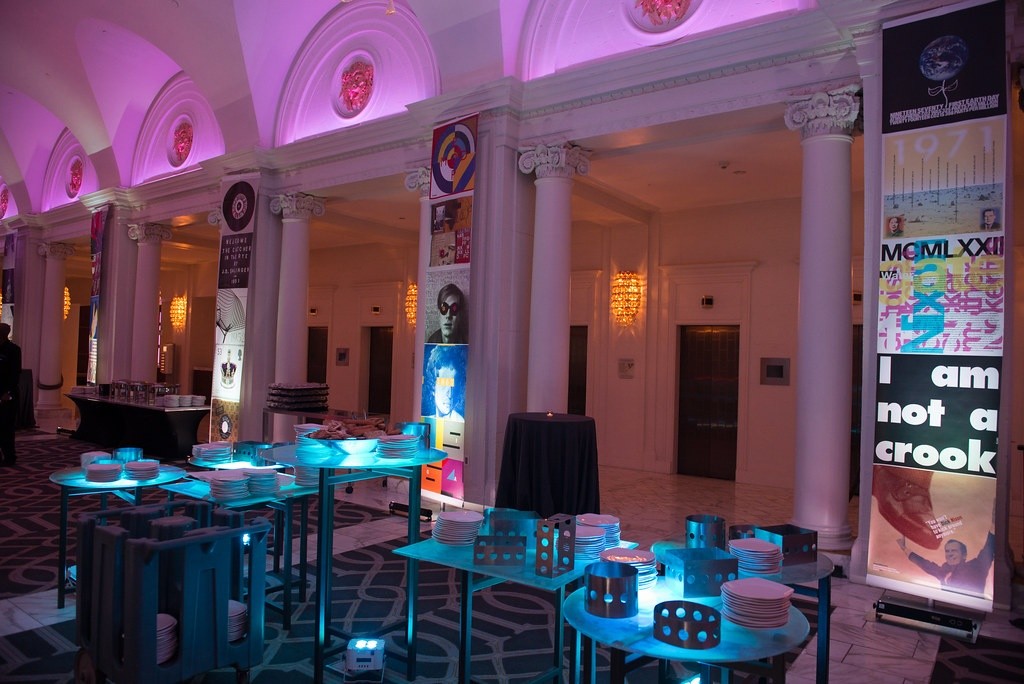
[350,409,366,421]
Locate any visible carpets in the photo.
[928,634,1024,684]
[0,530,837,684]
[15,427,56,437]
[0,438,393,600]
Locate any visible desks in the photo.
[390,523,639,684]
[187,449,294,556]
[650,535,835,684]
[495,412,600,529]
[63,393,210,461]
[259,444,449,649]
[262,407,389,495]
[563,576,811,684]
[15,369,36,429]
[157,471,319,631]
[49,464,188,610]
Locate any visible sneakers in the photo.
[872,465,943,551]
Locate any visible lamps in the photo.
[346,638,385,671]
[63,287,72,320]
[610,270,641,327]
[169,296,185,329]
[404,280,417,329]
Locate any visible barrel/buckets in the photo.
[400,422,430,449]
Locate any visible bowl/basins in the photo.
[304,432,379,456]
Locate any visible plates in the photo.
[431,510,485,546]
[293,466,322,486]
[207,469,280,500]
[556,526,606,560]
[227,599,249,642]
[179,394,192,406]
[293,424,328,456]
[728,538,784,574]
[85,464,122,481]
[124,460,159,480]
[719,577,795,630]
[155,613,178,664]
[191,443,231,462]
[164,395,179,407]
[375,435,420,458]
[191,395,205,407]
[599,547,658,589]
[575,513,620,549]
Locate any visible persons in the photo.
[896,497,996,594]
[885,217,902,237]
[420,282,468,424]
[0,322,22,467]
[981,209,1001,230]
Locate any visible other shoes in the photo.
[2,452,17,468]
